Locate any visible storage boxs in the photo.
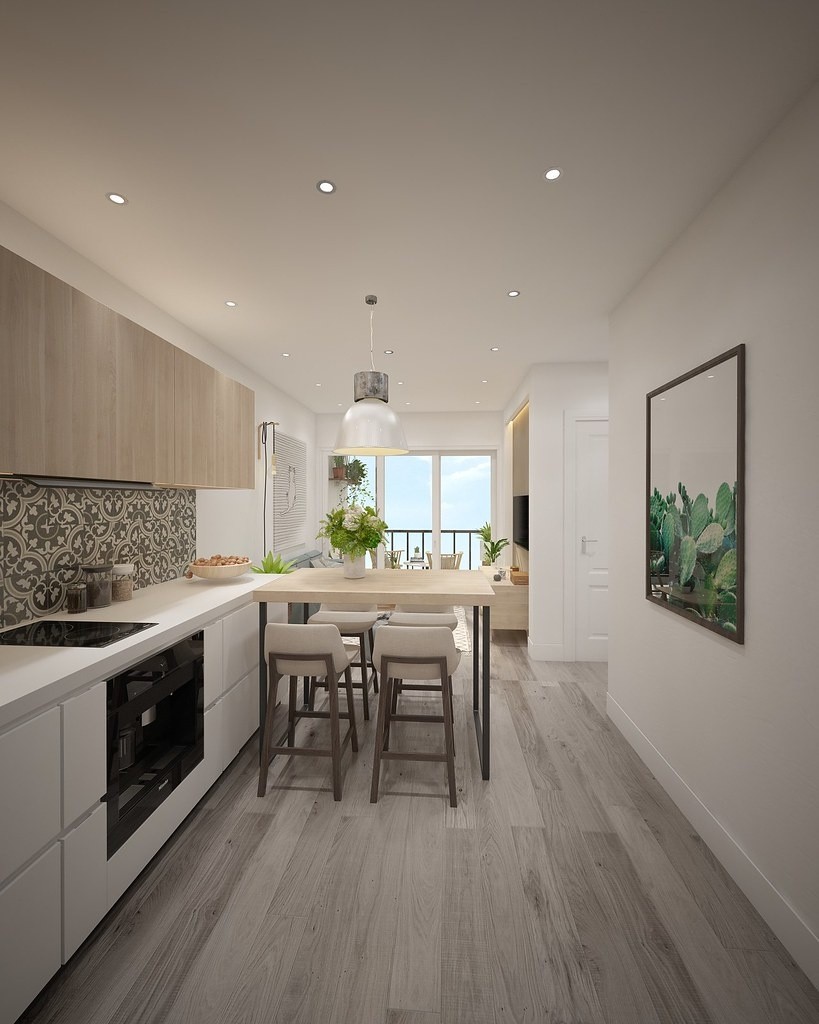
[510,571,529,585]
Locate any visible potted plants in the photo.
[477,522,510,570]
[414,546,420,558]
[332,456,347,478]
[346,459,368,484]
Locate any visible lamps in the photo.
[333,295,409,456]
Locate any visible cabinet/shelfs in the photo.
[0,245,257,490]
[0,602,288,1024]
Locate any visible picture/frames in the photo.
[644,343,746,645]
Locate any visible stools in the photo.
[388,605,458,723]
[256,623,359,802]
[307,603,379,721]
[370,625,461,808]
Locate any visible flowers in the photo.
[315,505,383,558]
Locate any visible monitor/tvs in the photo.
[513,495,529,551]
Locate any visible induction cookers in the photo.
[0,620,158,648]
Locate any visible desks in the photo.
[253,570,495,781]
[404,561,430,569]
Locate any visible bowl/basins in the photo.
[189,560,253,579]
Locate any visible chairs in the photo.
[369,549,463,569]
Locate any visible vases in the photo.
[344,553,366,579]
